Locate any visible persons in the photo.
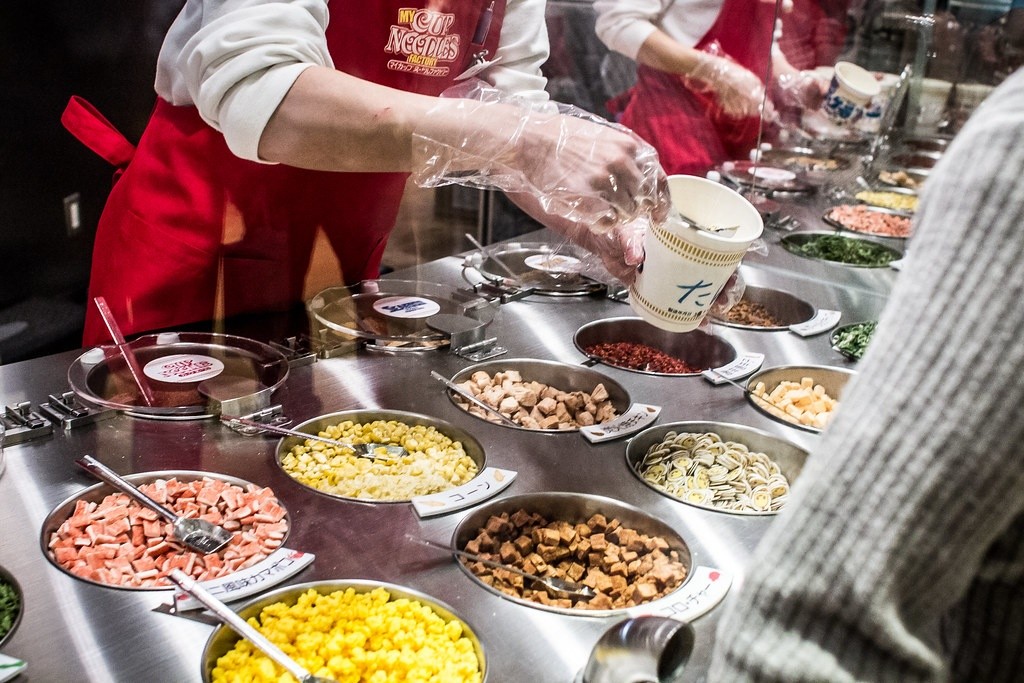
[777,0,836,69]
[60,0,671,350]
[595,0,830,180]
[704,65,1024,681]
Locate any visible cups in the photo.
[627,173,764,334]
[803,61,995,137]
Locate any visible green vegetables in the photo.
[787,234,896,265]
[832,321,877,359]
